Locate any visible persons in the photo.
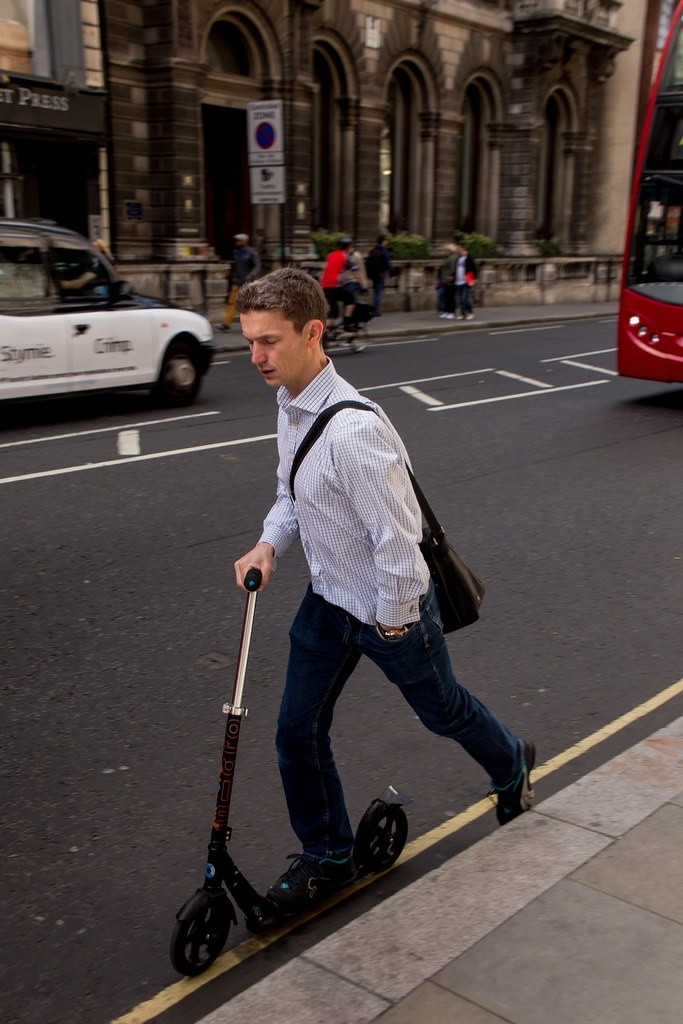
[231,269,536,918]
[435,242,477,320]
[90,238,120,276]
[365,234,393,316]
[320,238,369,347]
[216,233,262,332]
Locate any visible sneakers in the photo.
[266,846,358,910]
[497,740,536,826]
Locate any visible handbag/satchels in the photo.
[418,526,486,634]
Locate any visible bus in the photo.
[615,0,683,386]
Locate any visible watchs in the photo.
[379,624,406,641]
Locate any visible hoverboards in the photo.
[167,567,410,979]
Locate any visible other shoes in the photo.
[467,314,475,320]
[457,315,464,320]
[440,313,449,318]
[446,313,454,319]
[215,323,232,333]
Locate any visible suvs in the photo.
[0,217,216,408]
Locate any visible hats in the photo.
[234,234,248,242]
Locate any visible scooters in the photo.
[320,288,377,357]
